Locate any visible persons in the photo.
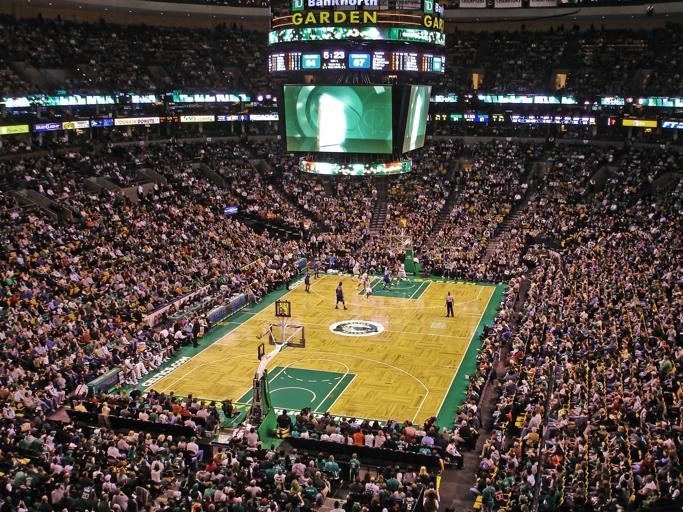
[2,10,683,511]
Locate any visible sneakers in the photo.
[314,277,320,279]
[383,287,391,290]
[335,306,348,310]
[305,289,311,294]
